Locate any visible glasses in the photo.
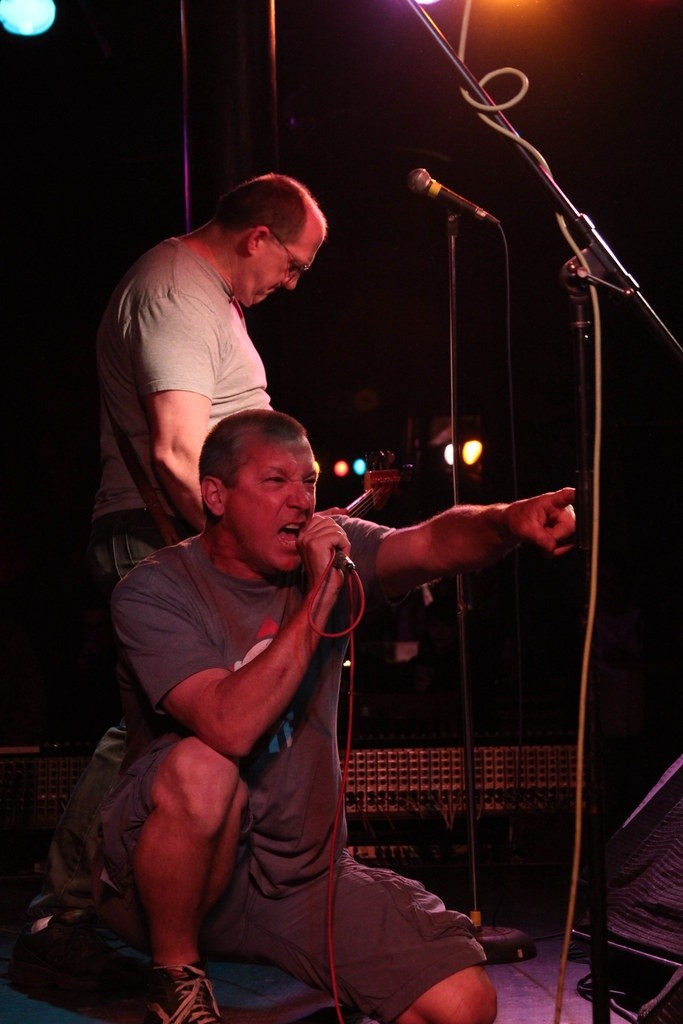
[251,224,312,282]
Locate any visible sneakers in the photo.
[8,920,147,992]
[144,962,224,1024]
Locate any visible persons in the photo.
[7,172,327,995]
[96,409,583,1024]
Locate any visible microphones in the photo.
[406,165,501,228]
[333,546,356,572]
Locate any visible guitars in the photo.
[343,447,406,518]
